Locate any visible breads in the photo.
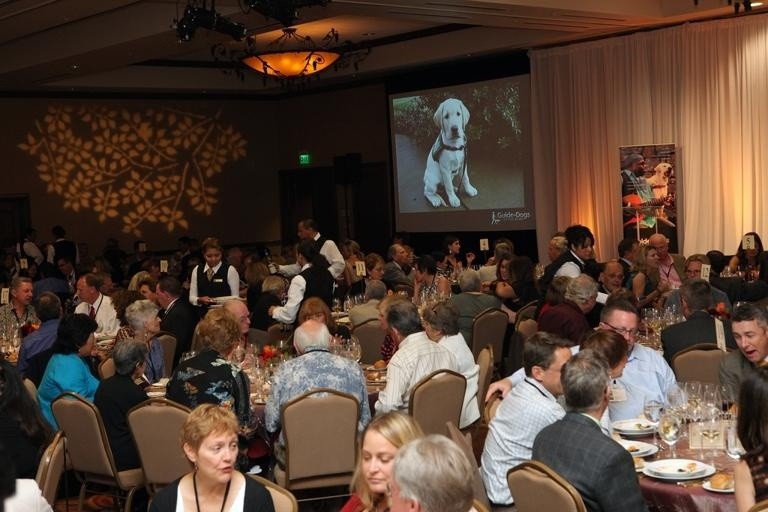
[366,359,386,380]
[710,473,734,489]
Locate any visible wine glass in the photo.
[642,380,745,462]
[9,334,21,353]
[330,297,343,320]
[640,303,684,350]
[250,367,265,399]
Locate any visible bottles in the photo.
[744,264,753,281]
[278,340,285,364]
[263,248,278,276]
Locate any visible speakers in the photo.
[332,153,362,184]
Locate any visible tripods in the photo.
[338,186,360,242]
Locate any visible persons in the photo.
[621,154,674,236]
[0,219,767,511]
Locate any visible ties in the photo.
[89,305,96,318]
[208,269,214,280]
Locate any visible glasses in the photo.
[604,320,639,336]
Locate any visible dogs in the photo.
[644,162,674,218]
[423,98,478,208]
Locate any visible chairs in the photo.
[0,255,767,512]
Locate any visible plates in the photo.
[617,439,660,459]
[641,458,721,480]
[366,365,389,371]
[611,418,656,438]
[254,399,270,404]
[702,480,735,494]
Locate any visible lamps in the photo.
[210,27,373,92]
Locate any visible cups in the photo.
[328,336,362,363]
